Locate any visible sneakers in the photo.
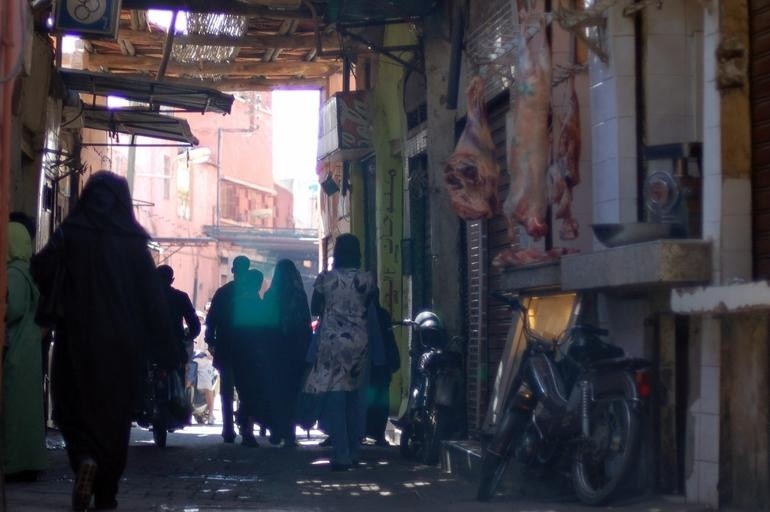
[221,433,391,472]
[94,482,118,510]
[70,459,96,512]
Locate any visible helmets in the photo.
[414,311,444,333]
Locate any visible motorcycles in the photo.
[135,330,218,450]
[478,290,652,503]
[388,312,478,464]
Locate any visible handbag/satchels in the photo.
[365,308,403,377]
